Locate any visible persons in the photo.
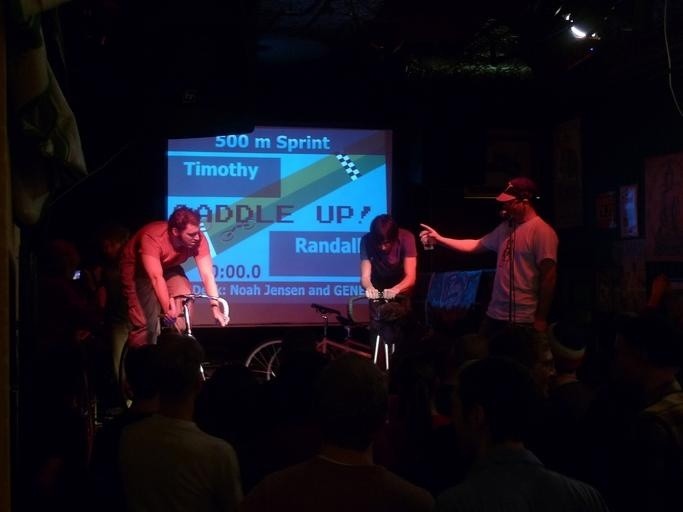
[13,308,683,512]
[102,223,136,382]
[419,175,560,339]
[117,206,234,347]
[42,239,110,355]
[356,213,420,314]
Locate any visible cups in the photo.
[423,233,435,251]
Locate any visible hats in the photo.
[496,178,536,202]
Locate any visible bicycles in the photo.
[116,289,405,419]
